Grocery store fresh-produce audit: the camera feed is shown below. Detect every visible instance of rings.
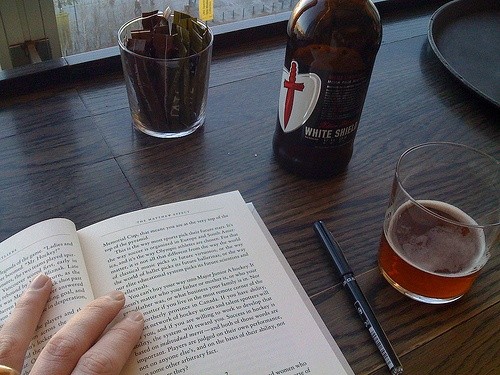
[0,364,21,375]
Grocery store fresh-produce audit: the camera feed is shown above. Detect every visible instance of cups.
[377,140,500,306]
[117,12,214,140]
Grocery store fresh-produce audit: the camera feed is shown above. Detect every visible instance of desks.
[1,0,500,375]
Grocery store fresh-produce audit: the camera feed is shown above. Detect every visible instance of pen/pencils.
[314,220,404,375]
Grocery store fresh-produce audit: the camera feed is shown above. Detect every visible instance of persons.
[0,273,146,375]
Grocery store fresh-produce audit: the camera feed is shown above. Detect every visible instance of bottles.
[272,0,383,180]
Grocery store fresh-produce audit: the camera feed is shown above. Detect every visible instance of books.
[0,189,354,375]
